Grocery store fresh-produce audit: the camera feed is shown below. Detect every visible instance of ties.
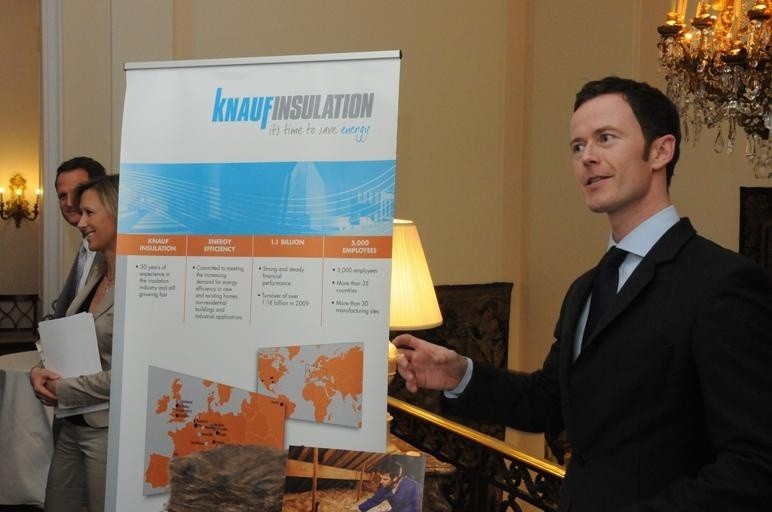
[71,244,87,296]
[583,246,626,345]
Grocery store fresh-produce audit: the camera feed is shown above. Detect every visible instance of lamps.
[0,172,43,229]
[654,1,772,179]
[389,217,443,388]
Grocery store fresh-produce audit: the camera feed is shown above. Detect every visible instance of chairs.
[0,295,40,352]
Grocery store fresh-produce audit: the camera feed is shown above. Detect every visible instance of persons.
[350,459,421,512]
[390,76,772,511]
[29,173,119,512]
[53,155,106,321]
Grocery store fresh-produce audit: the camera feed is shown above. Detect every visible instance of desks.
[0,347,53,507]
